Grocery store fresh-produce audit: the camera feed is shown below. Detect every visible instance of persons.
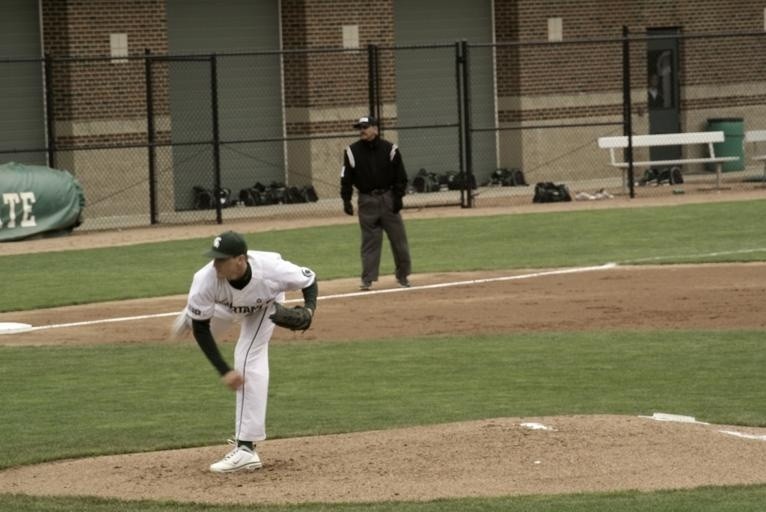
[171,226,318,475]
[340,118,411,291]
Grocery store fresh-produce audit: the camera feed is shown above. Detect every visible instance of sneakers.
[210,445,262,473]
[575,188,614,200]
[343,199,353,216]
[393,195,402,214]
[360,280,371,288]
[396,273,410,287]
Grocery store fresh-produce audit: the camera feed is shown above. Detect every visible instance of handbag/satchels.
[412,167,477,192]
[189,181,318,210]
[532,182,572,202]
[489,168,529,187]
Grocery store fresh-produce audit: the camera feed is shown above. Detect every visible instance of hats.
[201,231,247,259]
[352,117,377,127]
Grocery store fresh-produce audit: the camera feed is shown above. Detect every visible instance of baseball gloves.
[270,301,310,329]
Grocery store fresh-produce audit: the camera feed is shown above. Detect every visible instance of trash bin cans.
[708,117,745,172]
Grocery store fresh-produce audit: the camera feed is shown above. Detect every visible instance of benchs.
[598,131,738,196]
